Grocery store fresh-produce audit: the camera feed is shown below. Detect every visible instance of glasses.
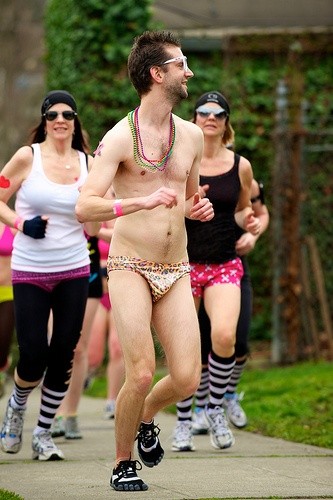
[158,56,189,71]
[43,111,78,121]
[195,105,229,119]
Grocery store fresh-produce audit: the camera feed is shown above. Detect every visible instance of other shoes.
[51,414,82,439]
[223,393,247,429]
[103,401,115,419]
[192,406,210,434]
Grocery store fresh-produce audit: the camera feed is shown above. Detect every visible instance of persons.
[0,91,101,461]
[171,91,263,450]
[74,31,214,491]
[0,180,270,439]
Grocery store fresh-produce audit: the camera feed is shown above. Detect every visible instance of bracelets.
[14,215,23,231]
[127,105,176,173]
[113,199,123,217]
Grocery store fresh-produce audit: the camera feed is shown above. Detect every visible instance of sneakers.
[202,401,235,450]
[109,451,149,491]
[31,429,65,461]
[171,420,195,451]
[1,398,24,454]
[134,418,164,468]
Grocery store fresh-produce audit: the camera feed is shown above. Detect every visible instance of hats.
[193,91,230,115]
[40,89,78,113]
